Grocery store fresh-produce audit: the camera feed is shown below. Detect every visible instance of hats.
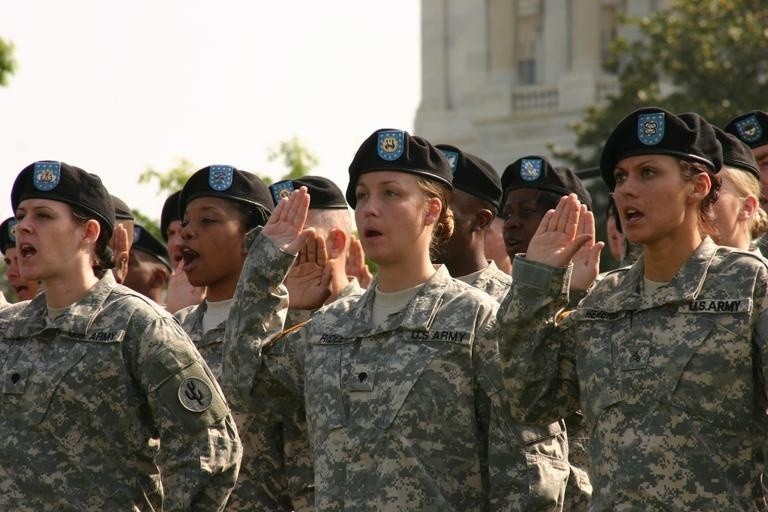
[131,224,173,273]
[599,106,724,192]
[495,154,593,219]
[160,190,182,243]
[177,164,275,219]
[109,194,134,220]
[722,109,768,150]
[10,160,116,258]
[345,128,456,211]
[0,217,18,256]
[434,144,503,210]
[709,124,762,181]
[268,175,348,210]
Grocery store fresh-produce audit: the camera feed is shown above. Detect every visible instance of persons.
[497,109,767,511]
[113,166,289,511]
[567,126,768,306]
[496,152,591,275]
[1,160,244,511]
[270,173,366,311]
[604,200,624,259]
[160,188,205,314]
[283,143,511,324]
[220,127,571,512]
[125,223,169,309]
[723,109,768,219]
[1,216,40,301]
[92,192,133,256]
[345,201,510,290]
